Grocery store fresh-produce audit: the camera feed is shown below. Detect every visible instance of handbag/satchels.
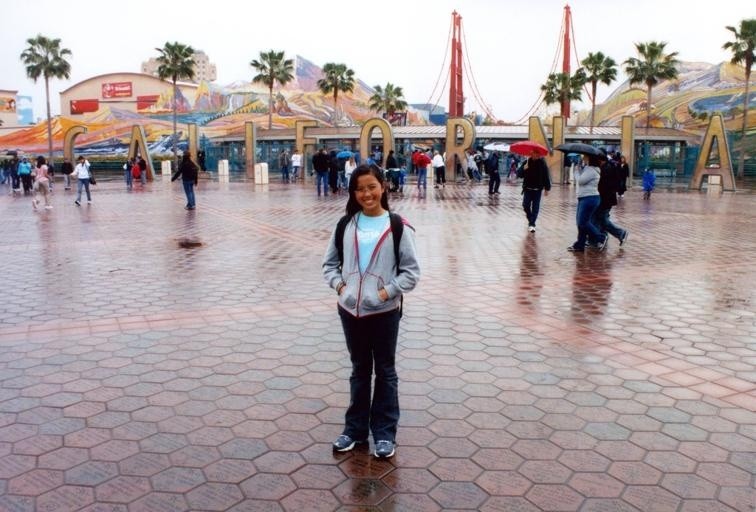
[89,175,96,184]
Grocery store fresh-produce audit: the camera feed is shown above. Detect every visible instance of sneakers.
[528,226,535,232]
[373,439,396,458]
[332,435,368,452]
[567,231,628,251]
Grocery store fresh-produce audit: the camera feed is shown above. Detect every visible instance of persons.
[322,162,421,458]
[126,157,136,189]
[462,148,523,194]
[197,148,207,172]
[69,156,92,207]
[280,141,447,198]
[517,150,552,231]
[137,153,147,187]
[562,152,631,251]
[171,150,198,209]
[0,152,74,210]
[641,166,656,200]
